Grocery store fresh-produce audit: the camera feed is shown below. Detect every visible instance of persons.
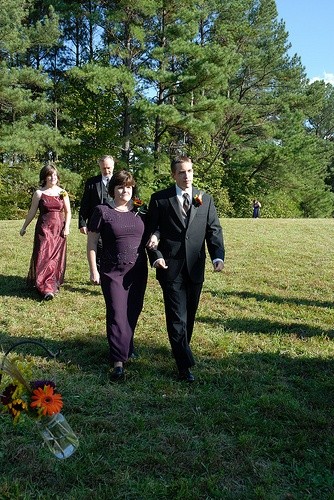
[252,199,261,218]
[145,156,225,381]
[78,155,115,271]
[20,165,72,301]
[86,170,160,378]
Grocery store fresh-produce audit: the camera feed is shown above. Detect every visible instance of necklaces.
[113,200,130,211]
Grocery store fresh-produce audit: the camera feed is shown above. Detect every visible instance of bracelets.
[22,229,26,231]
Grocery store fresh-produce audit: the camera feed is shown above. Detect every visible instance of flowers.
[193,192,204,207]
[59,190,66,197]
[0,352,63,425]
[133,197,147,215]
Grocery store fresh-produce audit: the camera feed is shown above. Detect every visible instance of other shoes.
[44,293,54,301]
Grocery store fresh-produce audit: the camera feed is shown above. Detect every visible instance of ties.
[182,192,190,216]
[105,178,109,188]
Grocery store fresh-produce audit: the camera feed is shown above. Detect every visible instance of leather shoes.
[178,367,195,381]
[110,362,125,382]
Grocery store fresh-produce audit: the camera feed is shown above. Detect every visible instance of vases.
[35,413,79,459]
